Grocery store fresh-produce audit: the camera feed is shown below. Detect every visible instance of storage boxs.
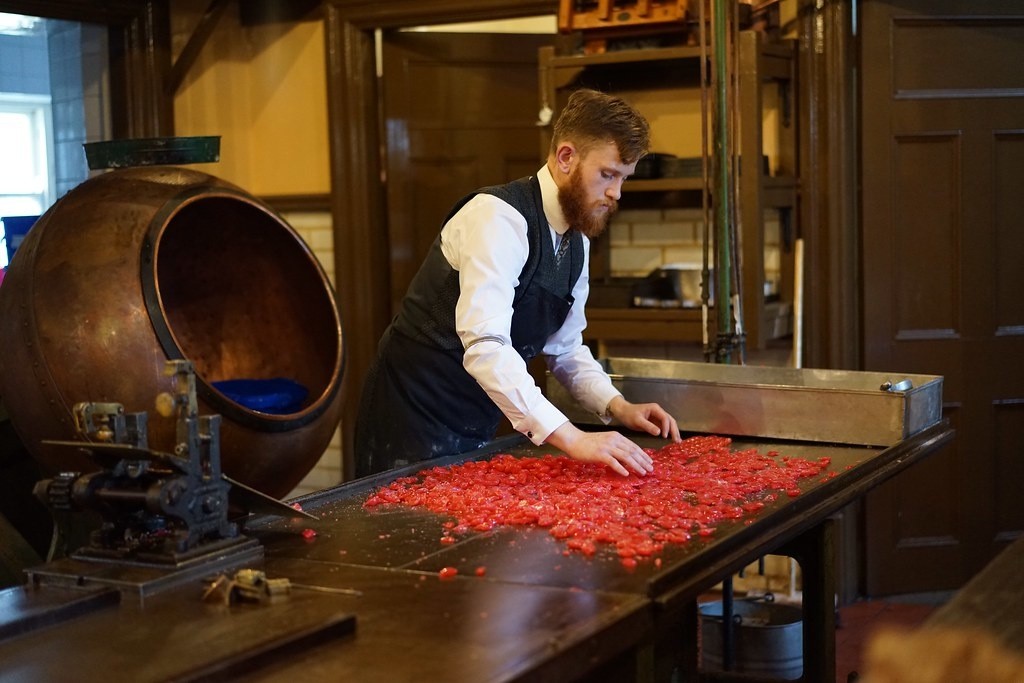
[543,357,943,447]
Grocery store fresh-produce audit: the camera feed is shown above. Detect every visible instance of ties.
[556,228,575,268]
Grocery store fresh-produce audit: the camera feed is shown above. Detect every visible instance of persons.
[352,90,682,477]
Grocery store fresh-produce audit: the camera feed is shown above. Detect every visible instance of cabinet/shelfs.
[535,31,795,352]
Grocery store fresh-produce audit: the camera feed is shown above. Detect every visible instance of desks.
[0,402,957,683]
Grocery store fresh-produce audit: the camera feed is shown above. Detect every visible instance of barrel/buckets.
[696,593,803,683]
[0,164,346,552]
